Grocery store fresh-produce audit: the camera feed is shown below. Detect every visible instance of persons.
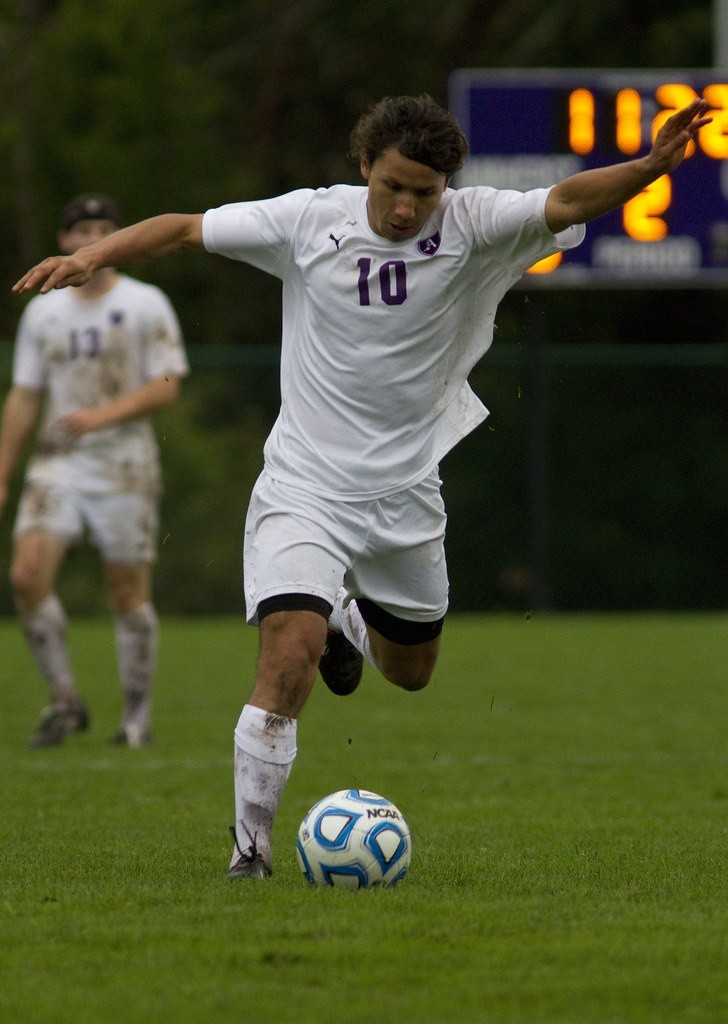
[0,194,189,747]
[12,94,712,882]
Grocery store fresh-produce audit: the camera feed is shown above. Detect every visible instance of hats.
[59,192,125,232]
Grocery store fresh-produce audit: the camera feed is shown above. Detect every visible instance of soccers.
[296,790,412,887]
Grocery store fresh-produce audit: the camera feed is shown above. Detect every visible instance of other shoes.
[28,699,90,749]
[105,731,153,749]
[227,819,272,883]
[318,627,364,696]
